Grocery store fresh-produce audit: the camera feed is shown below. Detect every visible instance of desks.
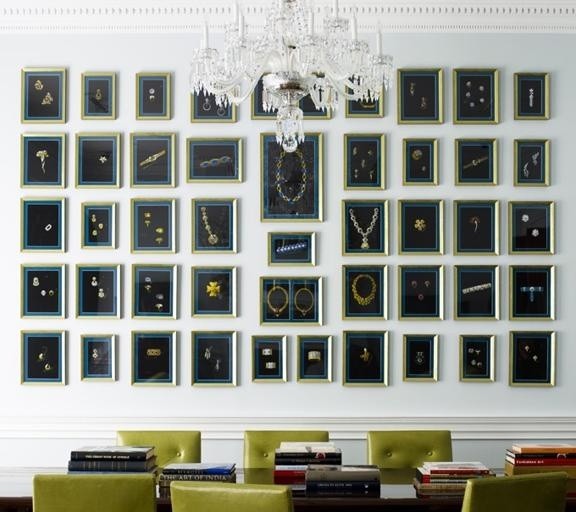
[1,464,505,510]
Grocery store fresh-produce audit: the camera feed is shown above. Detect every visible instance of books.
[503,441,575,500]
[159,462,236,499]
[412,461,496,500]
[274,442,343,497]
[68,446,158,474]
[305,465,381,499]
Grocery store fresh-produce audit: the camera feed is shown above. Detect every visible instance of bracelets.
[200,155,233,169]
[140,150,165,168]
[276,243,307,252]
[519,286,544,301]
[462,283,493,295]
[459,155,490,171]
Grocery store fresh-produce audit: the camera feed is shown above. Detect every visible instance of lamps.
[187,2,398,156]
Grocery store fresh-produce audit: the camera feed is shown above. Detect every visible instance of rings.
[262,349,273,356]
[146,348,162,357]
[307,351,324,360]
[265,362,277,370]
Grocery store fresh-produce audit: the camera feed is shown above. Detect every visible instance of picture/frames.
[401,137,440,188]
[257,130,326,225]
[187,70,241,124]
[507,263,558,323]
[399,332,441,384]
[130,262,180,321]
[190,196,240,255]
[455,332,496,384]
[257,274,326,328]
[506,329,558,388]
[128,130,178,190]
[19,69,68,124]
[450,69,502,127]
[395,198,447,258]
[73,262,123,321]
[73,130,123,191]
[78,332,118,383]
[249,70,334,122]
[126,196,179,256]
[249,333,290,384]
[510,70,552,122]
[340,263,390,322]
[185,136,245,184]
[343,74,387,121]
[395,262,447,323]
[451,262,502,323]
[512,137,552,189]
[189,263,241,321]
[19,130,68,191]
[78,199,119,252]
[396,70,446,126]
[78,70,118,122]
[133,70,174,122]
[266,230,318,268]
[19,328,67,388]
[342,132,388,193]
[18,194,68,254]
[129,328,179,389]
[294,333,335,384]
[339,196,392,259]
[451,198,502,258]
[454,136,500,188]
[188,329,239,388]
[505,199,558,258]
[340,329,391,389]
[18,261,69,322]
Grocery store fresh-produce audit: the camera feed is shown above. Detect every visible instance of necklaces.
[276,146,308,207]
[350,207,379,250]
[351,274,377,307]
[294,287,314,316]
[201,206,226,246]
[268,285,290,318]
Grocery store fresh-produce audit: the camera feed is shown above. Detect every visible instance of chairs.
[459,471,574,511]
[168,478,293,511]
[114,426,203,464]
[363,428,454,469]
[243,429,330,483]
[31,470,158,510]
